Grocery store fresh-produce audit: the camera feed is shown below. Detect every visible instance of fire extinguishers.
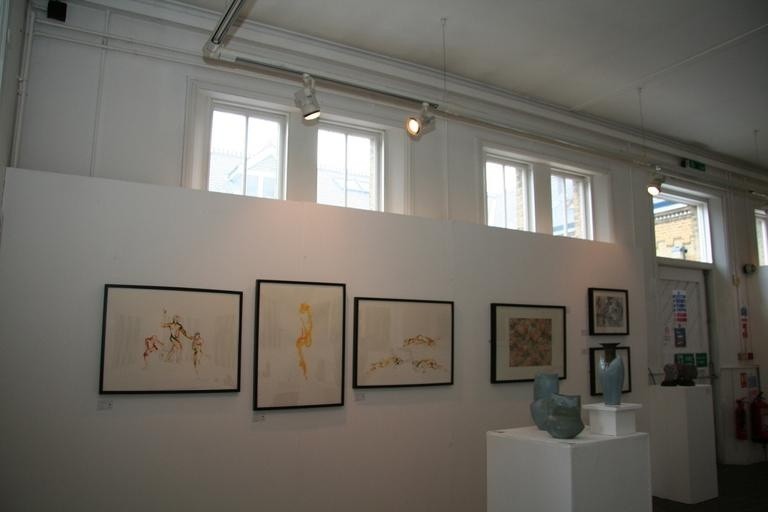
[734,391,768,443]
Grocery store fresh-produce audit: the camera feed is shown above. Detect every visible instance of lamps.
[407,101,434,136]
[647,165,664,196]
[295,72,320,122]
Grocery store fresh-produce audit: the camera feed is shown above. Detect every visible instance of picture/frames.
[252,280,347,411]
[352,296,454,389]
[589,346,631,398]
[588,287,630,338]
[489,301,568,385]
[100,284,243,396]
